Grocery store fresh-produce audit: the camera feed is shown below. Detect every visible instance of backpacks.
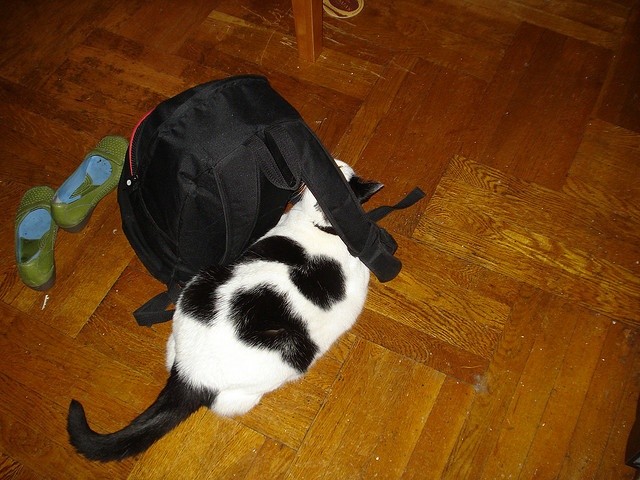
[117,74,428,327]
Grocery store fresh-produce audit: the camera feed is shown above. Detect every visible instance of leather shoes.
[14,187,58,292]
[53,135,129,234]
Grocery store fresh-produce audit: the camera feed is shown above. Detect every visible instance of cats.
[66,159,386,463]
